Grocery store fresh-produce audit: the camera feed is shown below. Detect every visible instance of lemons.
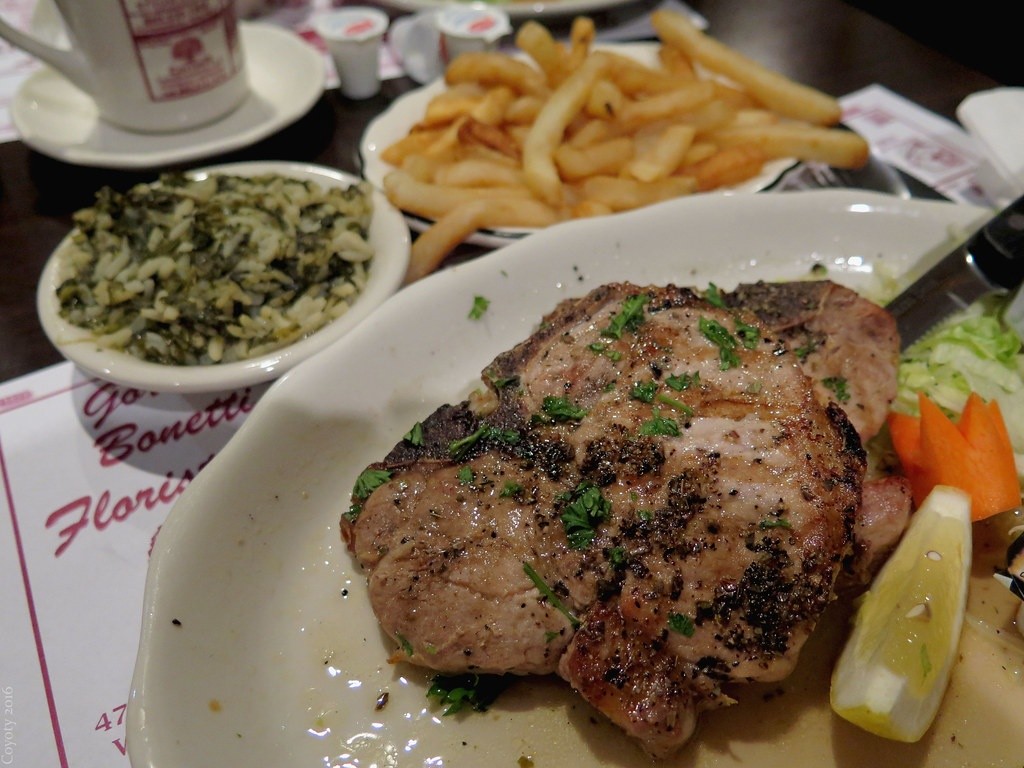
[829,484,972,742]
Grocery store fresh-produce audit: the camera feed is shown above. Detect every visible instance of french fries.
[382,12,871,286]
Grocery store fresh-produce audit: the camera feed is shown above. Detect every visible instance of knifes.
[883,192,1024,367]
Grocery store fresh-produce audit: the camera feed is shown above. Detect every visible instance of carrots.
[887,391,1021,523]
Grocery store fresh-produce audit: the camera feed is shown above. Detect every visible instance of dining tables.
[1,1,1023,764]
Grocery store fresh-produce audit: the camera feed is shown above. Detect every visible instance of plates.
[127,177,1021,766]
[32,157,411,392]
[358,36,814,246]
[10,21,331,171]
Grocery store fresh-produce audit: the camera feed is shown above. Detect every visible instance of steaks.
[339,279,913,761]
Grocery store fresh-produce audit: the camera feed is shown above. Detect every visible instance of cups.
[1,0,254,137]
